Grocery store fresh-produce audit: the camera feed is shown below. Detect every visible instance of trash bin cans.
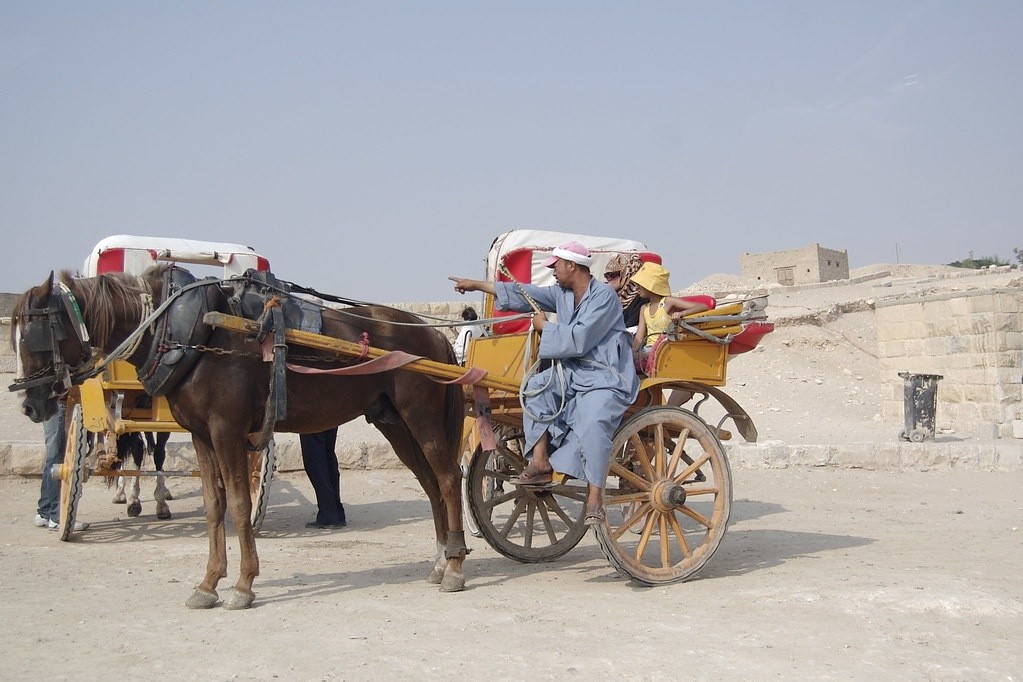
[897,371,944,442]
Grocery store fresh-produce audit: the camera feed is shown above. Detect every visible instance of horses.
[10,263,468,611]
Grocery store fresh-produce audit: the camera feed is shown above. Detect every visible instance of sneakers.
[48,518,90,530]
[35,515,49,526]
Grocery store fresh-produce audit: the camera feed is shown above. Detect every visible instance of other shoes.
[340,521,346,526]
[304,520,340,530]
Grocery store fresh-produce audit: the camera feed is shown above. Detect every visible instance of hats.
[630,262,671,296]
[543,240,591,268]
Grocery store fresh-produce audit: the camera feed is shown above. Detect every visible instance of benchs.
[648,295,717,377]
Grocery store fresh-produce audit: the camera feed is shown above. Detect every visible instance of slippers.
[509,466,552,484]
[584,506,606,525]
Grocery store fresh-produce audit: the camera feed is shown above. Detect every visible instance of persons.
[603,253,650,328]
[450,307,490,366]
[34,401,92,531]
[448,241,641,524]
[629,261,709,374]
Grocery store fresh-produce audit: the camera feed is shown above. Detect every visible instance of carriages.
[8,264,775,611]
[462,229,662,538]
[51,236,275,543]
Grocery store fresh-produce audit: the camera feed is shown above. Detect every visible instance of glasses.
[604,272,620,281]
